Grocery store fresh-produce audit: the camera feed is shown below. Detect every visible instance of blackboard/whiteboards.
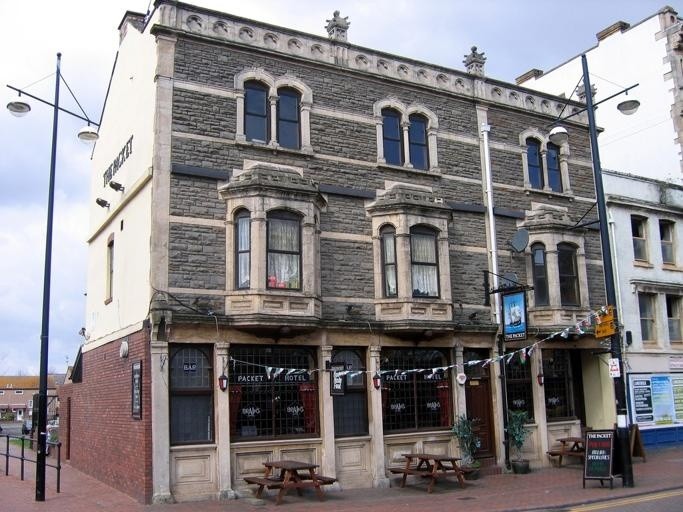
[131,360,142,421]
[584,429,614,480]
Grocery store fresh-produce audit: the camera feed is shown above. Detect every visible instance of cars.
[19,413,61,448]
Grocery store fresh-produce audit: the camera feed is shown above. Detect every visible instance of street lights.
[543,48,646,486]
[4,47,110,502]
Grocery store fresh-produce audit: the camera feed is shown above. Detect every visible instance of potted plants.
[502,406,539,474]
[449,410,483,482]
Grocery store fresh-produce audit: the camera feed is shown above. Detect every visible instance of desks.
[398,454,464,496]
[556,437,588,470]
[256,458,324,505]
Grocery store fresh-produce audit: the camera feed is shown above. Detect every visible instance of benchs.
[241,476,287,493]
[387,464,440,478]
[546,450,587,459]
[299,473,337,487]
[438,462,475,478]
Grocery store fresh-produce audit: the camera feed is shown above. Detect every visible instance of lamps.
[537,372,546,386]
[218,371,228,393]
[372,370,382,391]
